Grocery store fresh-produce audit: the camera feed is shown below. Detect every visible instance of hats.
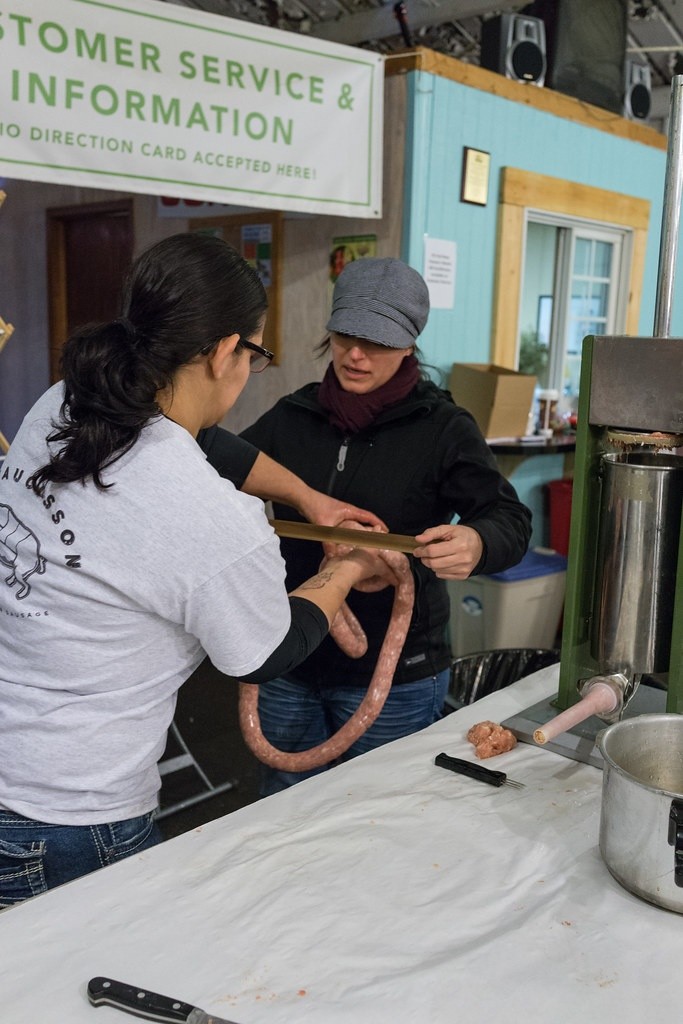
[325,256,430,349]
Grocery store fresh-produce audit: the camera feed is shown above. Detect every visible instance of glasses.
[202,334,275,374]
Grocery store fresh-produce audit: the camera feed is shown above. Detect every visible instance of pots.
[595,714,683,915]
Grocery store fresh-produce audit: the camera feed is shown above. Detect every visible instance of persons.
[0,232,398,911]
[236,258,536,799]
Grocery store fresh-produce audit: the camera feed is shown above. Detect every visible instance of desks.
[0,661,683,1024]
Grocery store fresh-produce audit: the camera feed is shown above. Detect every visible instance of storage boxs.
[451,363,538,439]
[448,545,568,658]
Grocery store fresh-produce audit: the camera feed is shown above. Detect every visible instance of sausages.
[237,521,414,771]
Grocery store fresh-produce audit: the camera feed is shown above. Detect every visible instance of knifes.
[87,977,240,1024]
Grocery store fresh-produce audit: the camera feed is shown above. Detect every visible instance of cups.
[538,389,558,439]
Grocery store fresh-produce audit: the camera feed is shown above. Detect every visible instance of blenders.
[500,335,683,770]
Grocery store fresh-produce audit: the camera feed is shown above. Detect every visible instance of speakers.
[622,60,653,123]
[519,0,627,114]
[480,14,548,88]
